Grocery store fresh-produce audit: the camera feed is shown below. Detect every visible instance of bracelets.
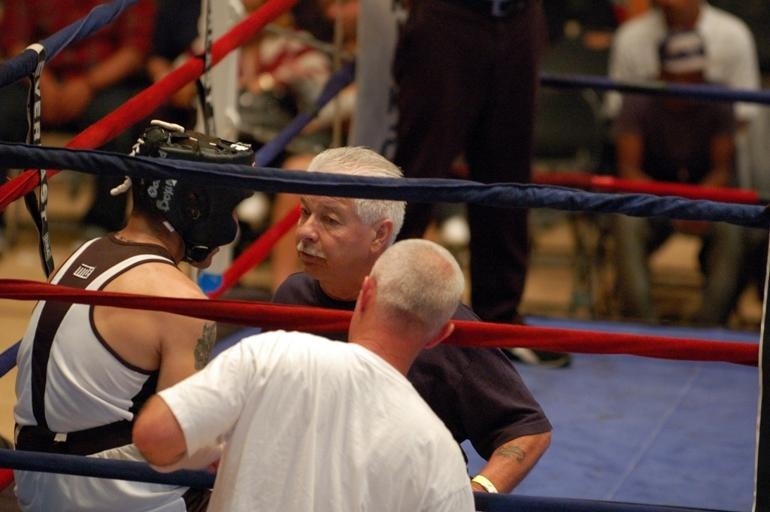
[472,475,498,494]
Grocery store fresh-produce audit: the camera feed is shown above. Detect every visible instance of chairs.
[531,91,612,318]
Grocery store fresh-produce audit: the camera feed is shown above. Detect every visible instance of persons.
[13,119,261,512]
[1,3,769,364]
[260,146,553,494]
[132,238,475,512]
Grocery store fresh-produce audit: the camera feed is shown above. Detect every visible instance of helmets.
[128,120,256,262]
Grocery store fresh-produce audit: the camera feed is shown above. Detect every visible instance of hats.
[660,30,708,76]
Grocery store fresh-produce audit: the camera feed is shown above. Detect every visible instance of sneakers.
[502,347,571,368]
